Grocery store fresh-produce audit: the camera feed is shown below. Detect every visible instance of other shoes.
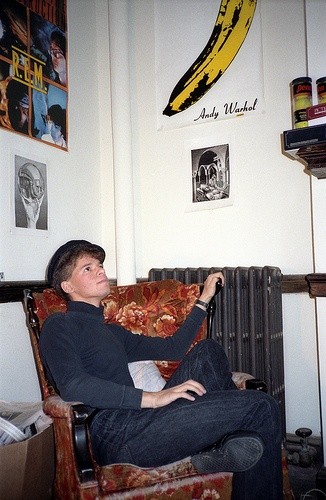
[192,431,264,474]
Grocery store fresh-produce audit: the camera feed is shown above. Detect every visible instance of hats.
[47,240,106,285]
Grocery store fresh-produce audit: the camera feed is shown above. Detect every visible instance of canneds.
[290,77,313,128]
[315,76,326,104]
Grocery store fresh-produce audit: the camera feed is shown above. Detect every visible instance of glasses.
[48,48,66,59]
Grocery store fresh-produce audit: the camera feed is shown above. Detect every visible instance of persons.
[0,1,66,148]
[38,239,283,500]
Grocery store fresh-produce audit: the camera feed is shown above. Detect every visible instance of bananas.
[162,0,257,114]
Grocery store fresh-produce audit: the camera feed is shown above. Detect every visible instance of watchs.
[193,297,209,309]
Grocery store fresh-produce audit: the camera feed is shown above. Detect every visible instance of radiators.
[149,266,287,450]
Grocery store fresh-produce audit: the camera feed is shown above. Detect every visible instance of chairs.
[21,279,292,500]
